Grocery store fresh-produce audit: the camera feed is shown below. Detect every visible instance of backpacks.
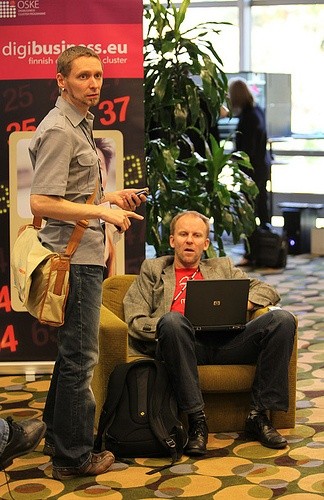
[93,357,188,459]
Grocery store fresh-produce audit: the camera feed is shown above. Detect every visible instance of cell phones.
[127,189,148,203]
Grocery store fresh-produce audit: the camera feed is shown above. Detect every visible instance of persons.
[121,210,296,458]
[92,137,112,191]
[227,78,274,268]
[27,46,149,481]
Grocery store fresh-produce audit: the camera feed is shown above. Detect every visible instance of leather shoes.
[52,450,115,480]
[182,410,209,456]
[244,412,288,449]
[43,443,55,455]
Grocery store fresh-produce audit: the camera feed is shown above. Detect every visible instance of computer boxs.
[283,208,316,256]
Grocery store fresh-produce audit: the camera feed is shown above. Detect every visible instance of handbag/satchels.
[11,221,70,328]
[242,224,288,270]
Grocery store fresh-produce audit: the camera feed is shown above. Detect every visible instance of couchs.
[84,276,298,432]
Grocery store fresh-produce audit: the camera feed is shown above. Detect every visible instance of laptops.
[184,278,251,333]
